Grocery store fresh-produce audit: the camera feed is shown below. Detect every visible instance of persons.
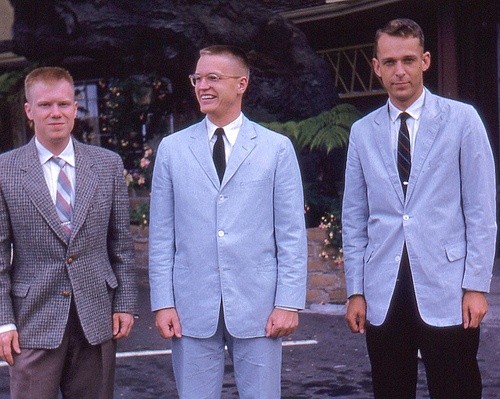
[148,45,308,399]
[342,19,498,399]
[0,67,138,399]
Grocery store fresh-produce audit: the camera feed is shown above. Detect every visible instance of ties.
[213,128,226,186]
[52,156,75,241]
[397,113,411,199]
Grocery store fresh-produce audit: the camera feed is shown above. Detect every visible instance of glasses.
[189,73,241,87]
